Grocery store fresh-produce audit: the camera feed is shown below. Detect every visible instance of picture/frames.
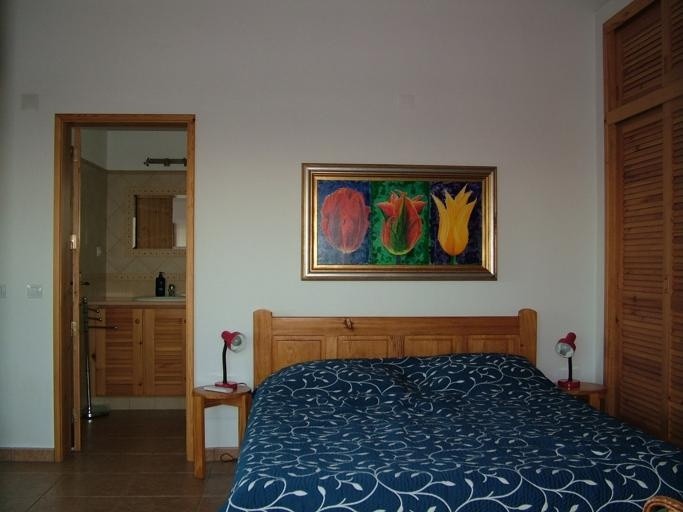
[298,163,498,283]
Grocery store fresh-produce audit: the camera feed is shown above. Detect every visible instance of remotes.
[204,384,234,394]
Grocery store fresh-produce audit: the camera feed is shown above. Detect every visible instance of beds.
[226,309,681,507]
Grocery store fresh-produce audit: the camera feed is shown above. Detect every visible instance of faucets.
[168,284,175,297]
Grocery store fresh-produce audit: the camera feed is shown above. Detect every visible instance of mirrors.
[133,193,187,251]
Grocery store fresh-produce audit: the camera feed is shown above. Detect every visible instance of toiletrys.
[155,271,166,296]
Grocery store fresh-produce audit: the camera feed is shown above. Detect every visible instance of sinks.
[133,295,186,301]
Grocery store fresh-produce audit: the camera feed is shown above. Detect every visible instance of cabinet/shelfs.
[88,306,185,398]
[598,1,683,445]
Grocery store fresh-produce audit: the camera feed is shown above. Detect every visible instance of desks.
[191,383,250,480]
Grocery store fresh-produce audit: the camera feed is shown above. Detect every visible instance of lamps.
[214,328,247,391]
[554,332,582,389]
